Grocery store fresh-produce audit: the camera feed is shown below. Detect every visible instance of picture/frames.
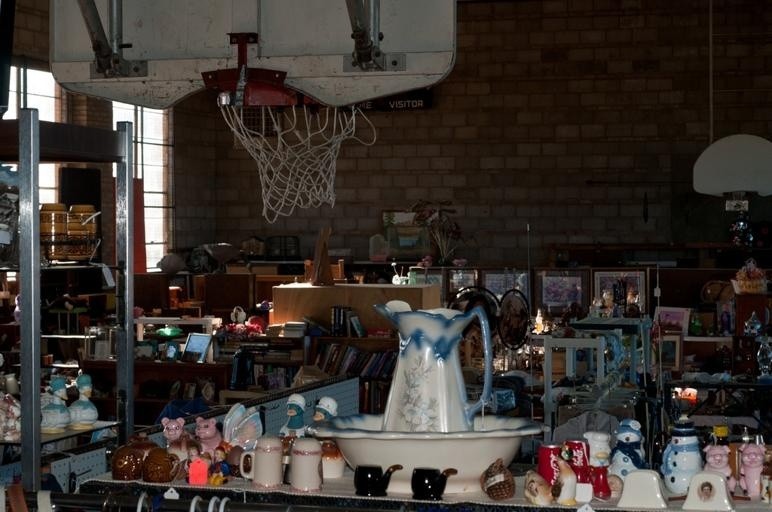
[182,332,212,363]
[409,266,651,316]
[653,306,691,336]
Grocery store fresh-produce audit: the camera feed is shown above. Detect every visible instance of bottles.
[311,227,335,286]
[4,373,19,395]
[84,325,117,360]
[40,203,98,260]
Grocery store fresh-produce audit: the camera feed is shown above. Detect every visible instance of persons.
[719,310,731,333]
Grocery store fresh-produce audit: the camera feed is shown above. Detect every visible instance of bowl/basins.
[304,415,552,494]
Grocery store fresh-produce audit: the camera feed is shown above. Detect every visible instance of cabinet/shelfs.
[539,334,605,441]
[254,275,305,325]
[1,108,133,495]
[234,333,400,417]
[193,272,254,325]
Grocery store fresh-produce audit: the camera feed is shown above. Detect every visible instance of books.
[221,304,401,415]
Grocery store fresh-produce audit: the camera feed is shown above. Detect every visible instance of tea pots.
[372,299,493,432]
[113,429,201,482]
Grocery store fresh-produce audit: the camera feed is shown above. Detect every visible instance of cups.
[239,437,345,494]
[407,272,417,285]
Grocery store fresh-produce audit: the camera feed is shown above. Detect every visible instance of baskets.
[730,279,767,294]
